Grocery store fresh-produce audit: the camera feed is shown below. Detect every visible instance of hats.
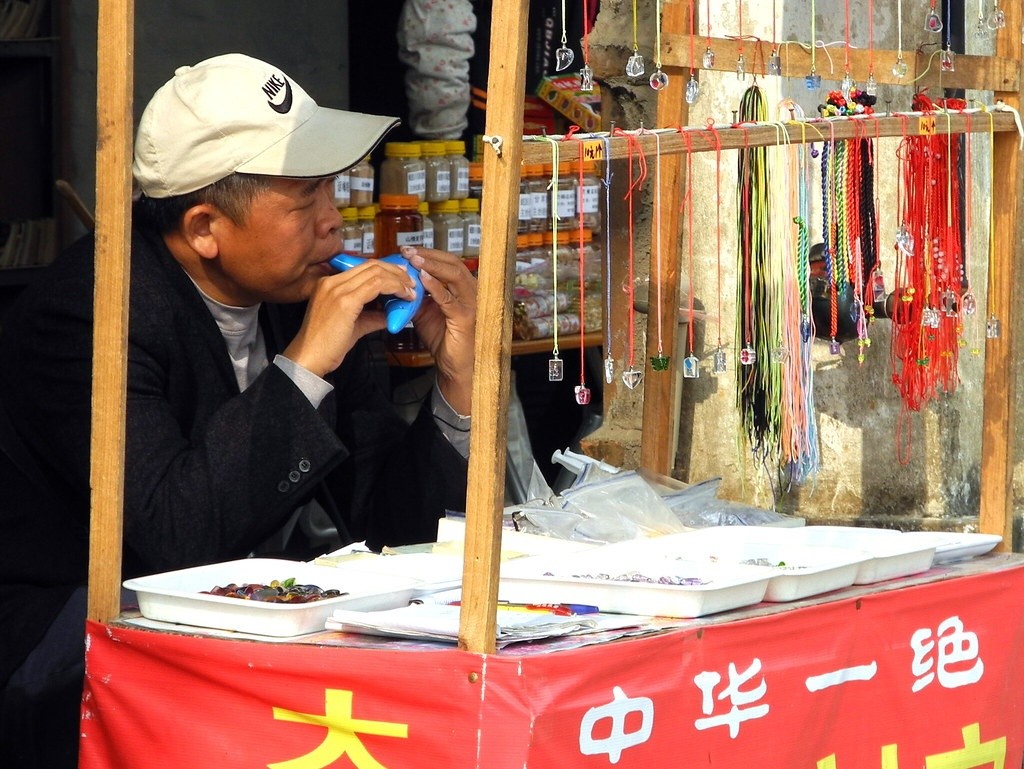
[132,52,400,199]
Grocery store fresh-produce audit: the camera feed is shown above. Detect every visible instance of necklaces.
[535,0,1004,407]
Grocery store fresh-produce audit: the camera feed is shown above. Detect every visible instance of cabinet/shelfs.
[1,0,74,308]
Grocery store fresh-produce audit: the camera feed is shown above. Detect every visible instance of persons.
[0,53,477,769]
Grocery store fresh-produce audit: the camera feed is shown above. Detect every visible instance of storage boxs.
[522,71,602,135]
